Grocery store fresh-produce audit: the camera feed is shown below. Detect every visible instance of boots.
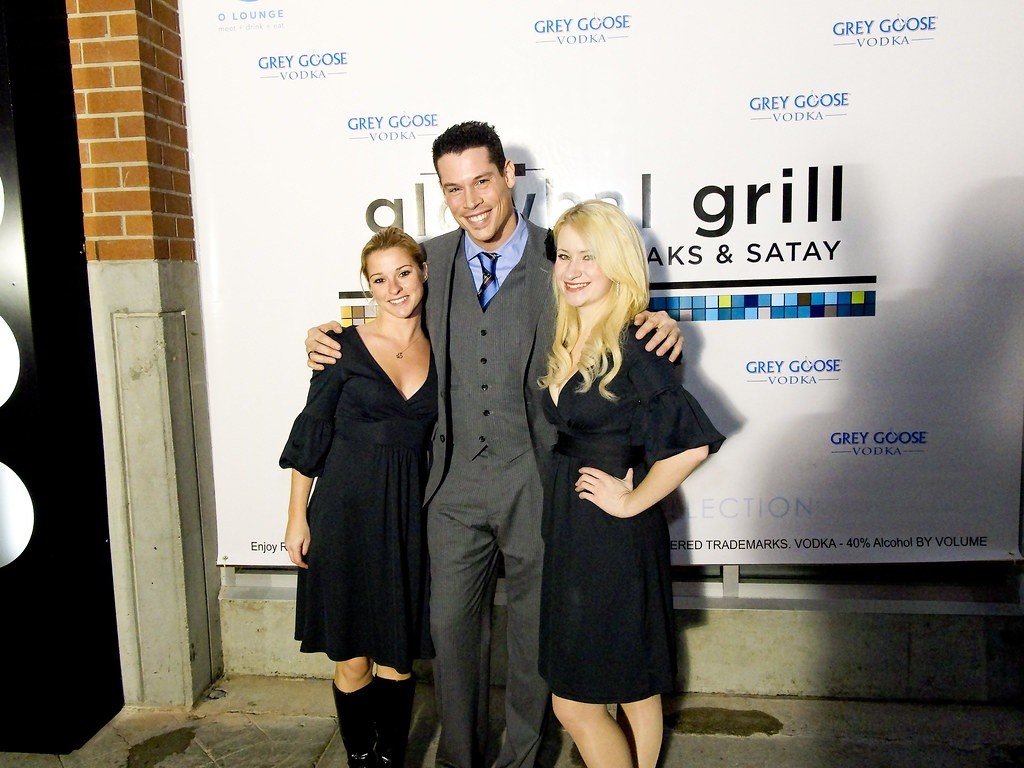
[374,673,417,768]
[332,676,376,768]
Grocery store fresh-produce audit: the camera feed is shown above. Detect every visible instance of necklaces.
[395,334,422,359]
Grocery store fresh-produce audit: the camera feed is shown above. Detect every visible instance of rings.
[308,351,312,358]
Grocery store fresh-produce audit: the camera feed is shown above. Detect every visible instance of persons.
[305,119,683,768]
[538,201,726,768]
[279,224,439,768]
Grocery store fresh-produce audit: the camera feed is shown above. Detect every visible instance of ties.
[477,251,502,313]
